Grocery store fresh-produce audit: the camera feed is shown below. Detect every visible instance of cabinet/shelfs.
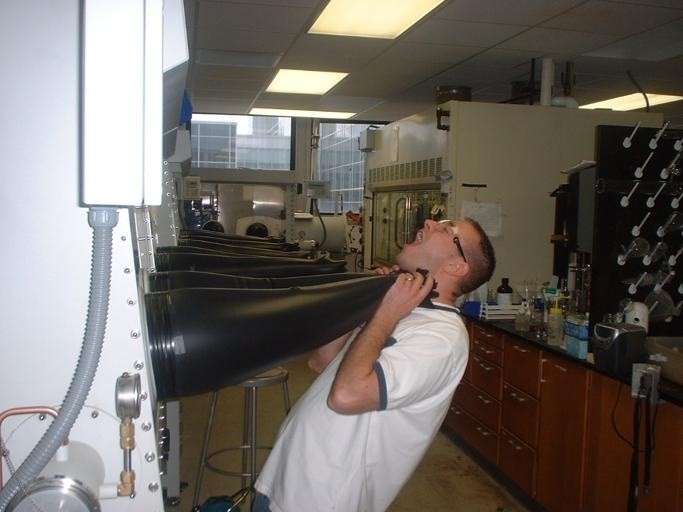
[497,330,543,512]
[439,316,502,486]
[534,346,588,511]
[580,370,682,511]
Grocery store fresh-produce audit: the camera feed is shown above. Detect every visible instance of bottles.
[497,277,569,346]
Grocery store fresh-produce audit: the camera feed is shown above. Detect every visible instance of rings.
[405,278,413,281]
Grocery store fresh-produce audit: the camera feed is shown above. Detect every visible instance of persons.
[246,215,497,511]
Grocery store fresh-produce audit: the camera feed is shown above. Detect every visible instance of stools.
[191,364,290,511]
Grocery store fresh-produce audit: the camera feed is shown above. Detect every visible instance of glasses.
[438,219,467,264]
